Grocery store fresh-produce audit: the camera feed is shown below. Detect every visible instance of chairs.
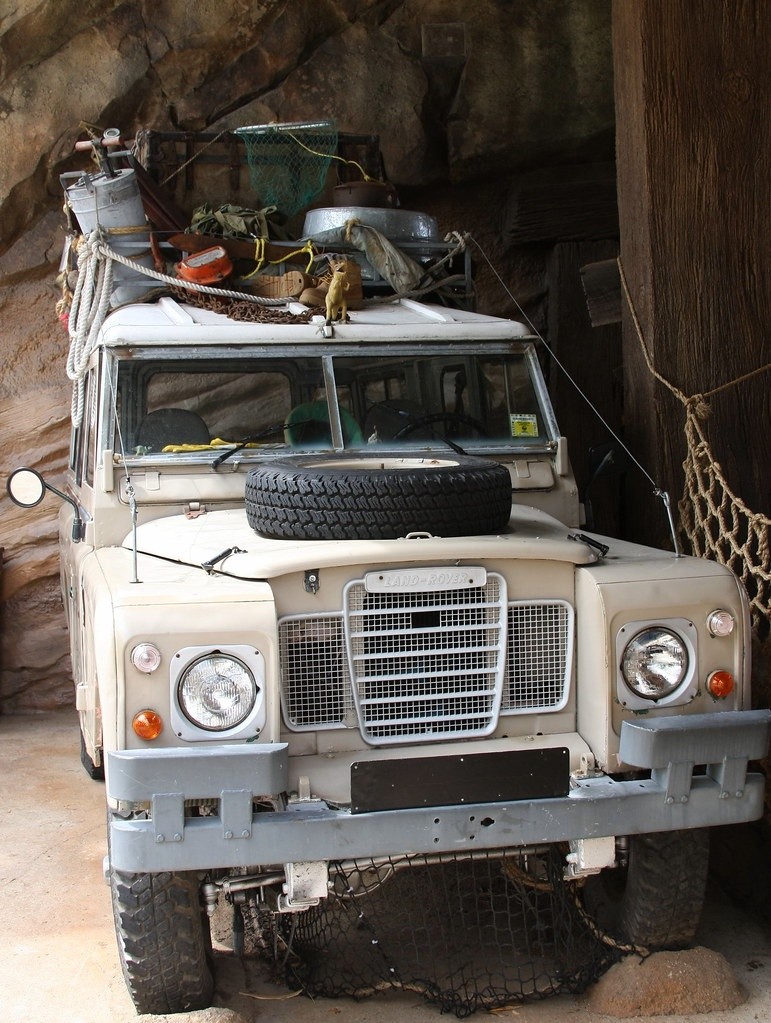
[360,400,434,444]
[134,407,209,453]
[286,397,362,450]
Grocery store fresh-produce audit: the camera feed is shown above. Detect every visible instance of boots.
[300,259,364,308]
[252,271,320,298]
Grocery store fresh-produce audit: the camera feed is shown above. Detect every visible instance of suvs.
[7,297,771,1014]
